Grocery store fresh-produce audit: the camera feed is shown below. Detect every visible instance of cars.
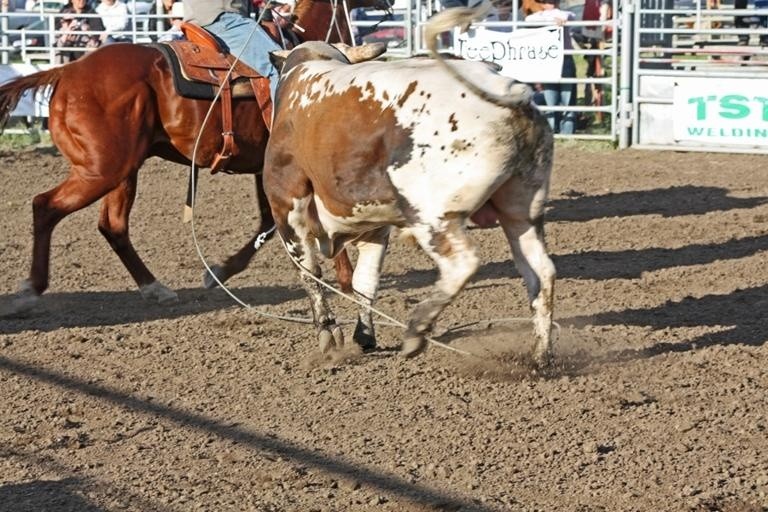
[349,0,607,49]
[672,0,768,47]
[0,0,158,58]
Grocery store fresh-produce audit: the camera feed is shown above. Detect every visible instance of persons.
[183,0,285,133]
[734,0,768,58]
[26,0,184,64]
[490,1,580,136]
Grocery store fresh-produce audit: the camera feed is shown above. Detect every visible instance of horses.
[0,0,395,306]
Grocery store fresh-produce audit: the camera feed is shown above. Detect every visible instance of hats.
[165,2,185,18]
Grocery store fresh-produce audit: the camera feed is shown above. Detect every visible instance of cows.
[262,5,557,378]
